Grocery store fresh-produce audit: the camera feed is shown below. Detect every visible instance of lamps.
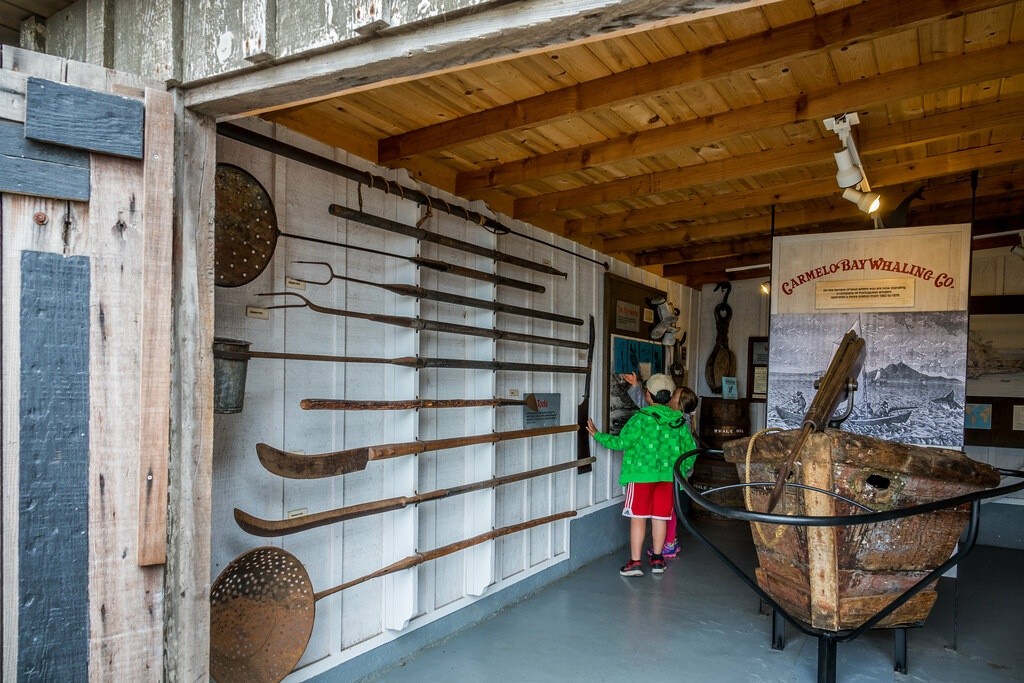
[840,186,881,215]
[833,144,863,189]
[1010,244,1024,260]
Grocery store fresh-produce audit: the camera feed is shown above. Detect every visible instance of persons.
[621,372,700,557]
[586,373,698,577]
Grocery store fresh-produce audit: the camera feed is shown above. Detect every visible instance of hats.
[646,373,676,398]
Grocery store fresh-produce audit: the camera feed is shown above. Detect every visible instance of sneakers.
[620,559,644,577]
[675,539,681,553]
[646,543,676,558]
[650,554,667,573]
